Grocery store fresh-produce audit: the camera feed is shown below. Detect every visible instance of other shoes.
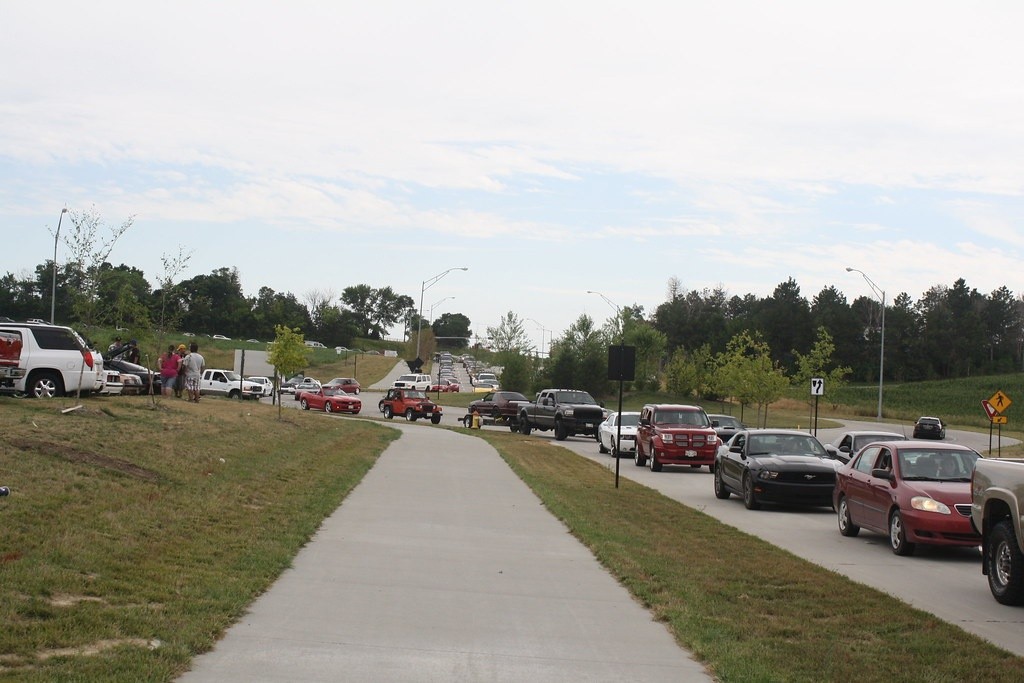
[185,398,193,402]
[193,398,199,403]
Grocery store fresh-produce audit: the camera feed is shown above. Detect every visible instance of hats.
[177,344,186,351]
[130,339,137,345]
[116,337,121,341]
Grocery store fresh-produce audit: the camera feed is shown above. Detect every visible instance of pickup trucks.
[970,456,1022,605]
[196,369,264,401]
[518,388,606,441]
[300,389,362,412]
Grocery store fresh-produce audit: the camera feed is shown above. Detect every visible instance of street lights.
[845,267,887,419]
[50,208,68,323]
[429,297,455,328]
[587,290,625,342]
[416,267,470,367]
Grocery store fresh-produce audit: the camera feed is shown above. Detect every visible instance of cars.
[432,354,499,394]
[824,430,911,467]
[322,377,362,395]
[181,329,379,355]
[712,428,849,512]
[392,373,432,394]
[829,442,988,555]
[246,375,321,395]
[638,404,722,469]
[468,391,531,418]
[99,359,163,396]
[383,387,443,424]
[913,415,946,440]
[705,414,744,445]
[598,410,644,457]
[0,320,106,403]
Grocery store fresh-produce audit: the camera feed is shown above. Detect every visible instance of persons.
[347,380,351,384]
[158,342,206,403]
[128,340,140,366]
[787,440,801,454]
[217,374,225,381]
[307,379,311,382]
[108,336,124,360]
[942,454,958,477]
[91,341,98,349]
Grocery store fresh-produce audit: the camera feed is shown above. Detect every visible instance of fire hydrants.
[471,410,480,429]
